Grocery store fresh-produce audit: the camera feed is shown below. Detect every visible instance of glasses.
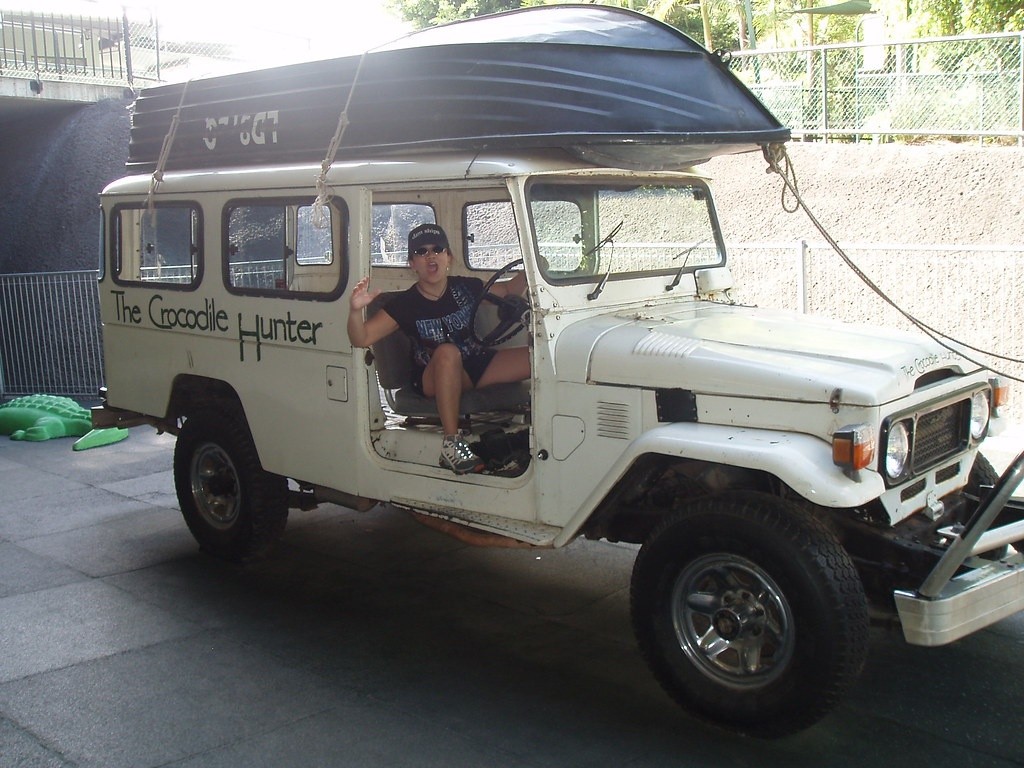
[411,246,447,257]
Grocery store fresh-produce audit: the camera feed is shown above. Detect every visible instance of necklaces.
[418,280,448,298]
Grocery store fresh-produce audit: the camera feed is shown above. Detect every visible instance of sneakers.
[439,433,485,475]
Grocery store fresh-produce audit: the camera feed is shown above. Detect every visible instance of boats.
[124,4,793,170]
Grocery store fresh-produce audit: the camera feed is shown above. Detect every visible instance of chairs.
[373,292,533,434]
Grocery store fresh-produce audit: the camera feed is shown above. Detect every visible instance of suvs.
[90,156,1024,739]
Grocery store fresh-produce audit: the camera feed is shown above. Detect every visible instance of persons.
[346,224,550,475]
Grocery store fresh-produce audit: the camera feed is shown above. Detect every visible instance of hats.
[408,224,449,254]
